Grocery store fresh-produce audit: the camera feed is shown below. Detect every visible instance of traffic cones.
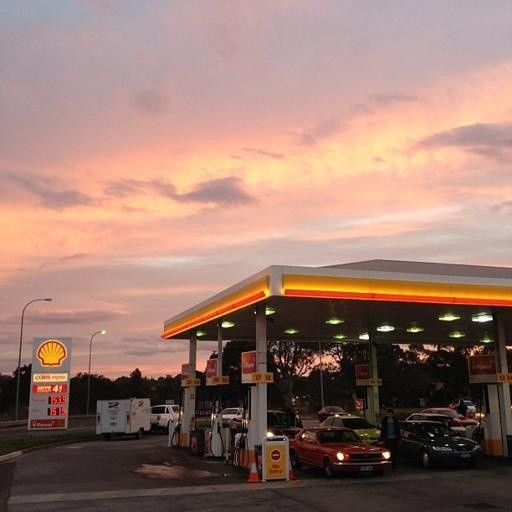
[246,456,263,483]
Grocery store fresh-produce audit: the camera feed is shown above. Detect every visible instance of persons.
[381,409,400,474]
[449,399,468,417]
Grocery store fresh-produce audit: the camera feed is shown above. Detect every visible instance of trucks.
[94,397,152,440]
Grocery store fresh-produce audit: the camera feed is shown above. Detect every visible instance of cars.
[211,407,246,422]
[317,406,351,422]
[405,397,479,437]
[320,415,381,440]
[396,420,481,468]
[267,410,303,437]
[151,404,184,428]
[285,426,392,476]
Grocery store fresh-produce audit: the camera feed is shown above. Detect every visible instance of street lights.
[87,330,106,416]
[16,298,53,421]
